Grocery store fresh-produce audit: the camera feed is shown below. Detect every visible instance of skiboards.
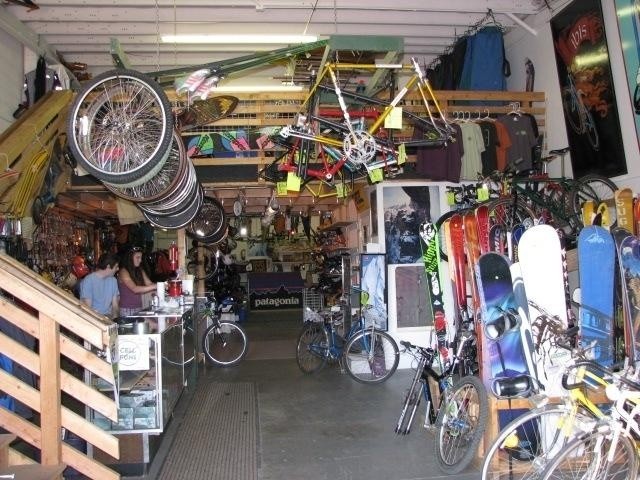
[419,206,503,437]
[583,188,640,236]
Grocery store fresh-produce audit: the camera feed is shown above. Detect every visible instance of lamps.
[158,1,321,43]
[205,85,304,93]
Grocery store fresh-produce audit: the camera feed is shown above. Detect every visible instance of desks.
[82,303,196,435]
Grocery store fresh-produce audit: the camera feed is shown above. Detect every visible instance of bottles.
[179,294,185,306]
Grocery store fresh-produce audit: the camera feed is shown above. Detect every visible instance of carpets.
[156,380,261,480]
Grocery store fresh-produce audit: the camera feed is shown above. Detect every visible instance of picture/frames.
[548,0,640,185]
[359,252,389,332]
[246,272,303,311]
[249,259,267,272]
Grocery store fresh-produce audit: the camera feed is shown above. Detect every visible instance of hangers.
[428,10,508,73]
[448,105,527,128]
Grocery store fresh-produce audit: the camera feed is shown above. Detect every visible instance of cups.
[152,296,159,308]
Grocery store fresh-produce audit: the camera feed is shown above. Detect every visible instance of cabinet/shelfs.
[318,221,352,253]
[271,237,315,265]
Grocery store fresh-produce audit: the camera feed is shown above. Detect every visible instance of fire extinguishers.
[168,240,178,271]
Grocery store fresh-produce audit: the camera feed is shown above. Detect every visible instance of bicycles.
[160,290,249,369]
[296,286,400,384]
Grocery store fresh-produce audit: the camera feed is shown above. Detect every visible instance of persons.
[79,253,120,320]
[117,245,168,317]
[187,262,197,275]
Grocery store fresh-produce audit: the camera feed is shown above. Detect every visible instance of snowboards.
[475,225,640,460]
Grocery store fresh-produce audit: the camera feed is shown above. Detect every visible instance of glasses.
[132,247,142,251]
